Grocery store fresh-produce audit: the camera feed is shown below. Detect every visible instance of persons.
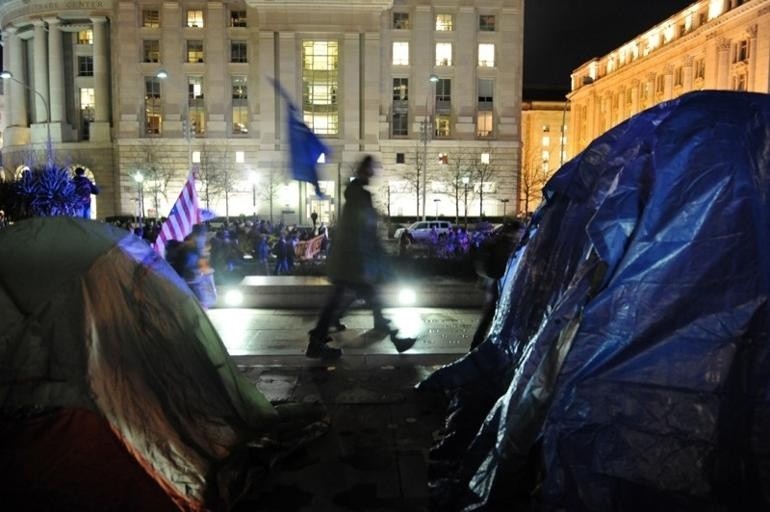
[329,287,391,331]
[71,167,99,220]
[101,212,330,306]
[400,225,493,266]
[306,154,417,358]
[470,220,526,353]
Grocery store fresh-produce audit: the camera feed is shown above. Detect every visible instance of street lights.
[151,71,168,136]
[0,70,52,167]
[560,76,594,168]
[422,75,440,221]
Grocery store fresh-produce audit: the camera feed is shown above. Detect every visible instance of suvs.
[394,220,453,246]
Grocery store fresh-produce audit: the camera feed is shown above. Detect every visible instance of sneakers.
[306,343,341,359]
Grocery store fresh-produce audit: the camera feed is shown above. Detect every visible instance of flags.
[269,77,332,197]
[154,169,215,260]
